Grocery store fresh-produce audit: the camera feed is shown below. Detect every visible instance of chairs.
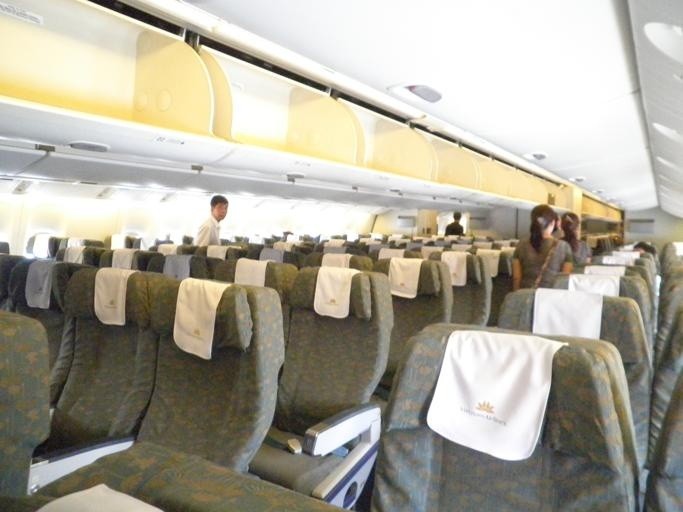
[451,244,476,254]
[106,235,135,249]
[302,252,374,271]
[8,258,92,406]
[377,258,451,399]
[590,255,651,269]
[195,245,248,259]
[358,234,519,246]
[466,248,513,327]
[0,312,51,494]
[602,250,653,261]
[133,280,285,477]
[269,242,312,253]
[377,247,423,260]
[316,242,366,255]
[249,265,393,510]
[246,248,304,267]
[491,288,651,496]
[427,249,490,329]
[552,274,651,345]
[55,239,104,248]
[147,255,209,280]
[149,244,199,255]
[210,260,296,347]
[0,442,350,511]
[56,247,105,266]
[651,239,683,512]
[50,267,162,447]
[412,246,451,259]
[26,235,60,258]
[370,322,640,511]
[100,249,162,271]
[613,240,659,251]
[571,264,653,298]
[133,238,174,249]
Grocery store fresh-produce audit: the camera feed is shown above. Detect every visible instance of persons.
[192,195,228,244]
[512,205,572,291]
[633,242,661,296]
[444,212,464,235]
[558,213,587,265]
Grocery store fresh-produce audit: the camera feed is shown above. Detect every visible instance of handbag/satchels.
[532,237,559,289]
